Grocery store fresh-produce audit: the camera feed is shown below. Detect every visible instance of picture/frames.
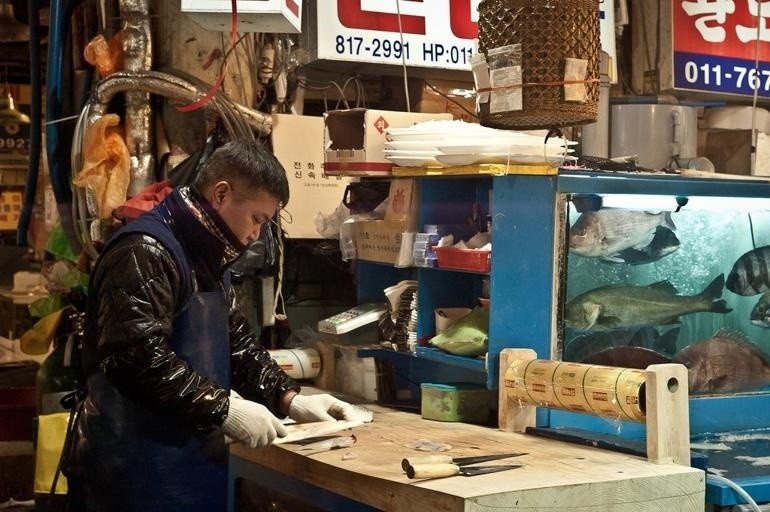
[0,185,26,239]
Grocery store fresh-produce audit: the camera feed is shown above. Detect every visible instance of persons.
[66,136,374,512]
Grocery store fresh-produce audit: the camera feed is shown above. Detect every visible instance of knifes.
[405,464,522,480]
[402,451,528,468]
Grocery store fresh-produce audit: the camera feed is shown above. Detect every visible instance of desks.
[230,386,707,512]
[0,363,41,442]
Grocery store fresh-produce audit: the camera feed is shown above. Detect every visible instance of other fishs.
[582,347,671,370]
[563,328,634,369]
[565,273,733,334]
[727,214,770,296]
[568,207,677,264]
[750,292,770,328]
[628,326,680,355]
[673,328,770,393]
[608,225,681,265]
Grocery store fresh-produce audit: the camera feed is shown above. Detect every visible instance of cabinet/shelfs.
[349,176,567,429]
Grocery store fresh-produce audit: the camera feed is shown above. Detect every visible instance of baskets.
[477,0,600,129]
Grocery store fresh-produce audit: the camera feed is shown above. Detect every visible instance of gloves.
[221,390,288,449]
[289,394,373,422]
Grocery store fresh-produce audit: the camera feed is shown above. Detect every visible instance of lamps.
[0,43,32,124]
[0,0,31,43]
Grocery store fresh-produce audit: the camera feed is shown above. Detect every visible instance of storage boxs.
[416,382,493,424]
[353,178,419,264]
[323,108,454,177]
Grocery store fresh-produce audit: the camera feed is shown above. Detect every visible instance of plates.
[382,125,579,166]
